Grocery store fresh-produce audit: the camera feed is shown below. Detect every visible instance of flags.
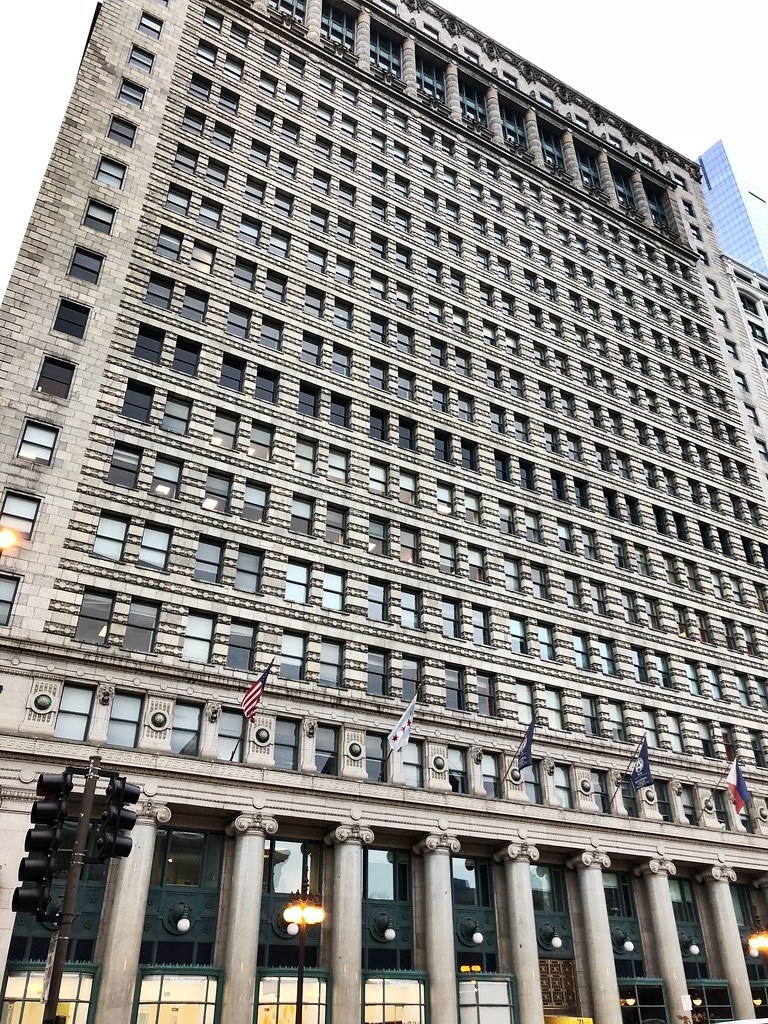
[631,740,654,790]
[726,757,752,816]
[388,693,418,751]
[241,669,268,722]
[518,721,533,769]
